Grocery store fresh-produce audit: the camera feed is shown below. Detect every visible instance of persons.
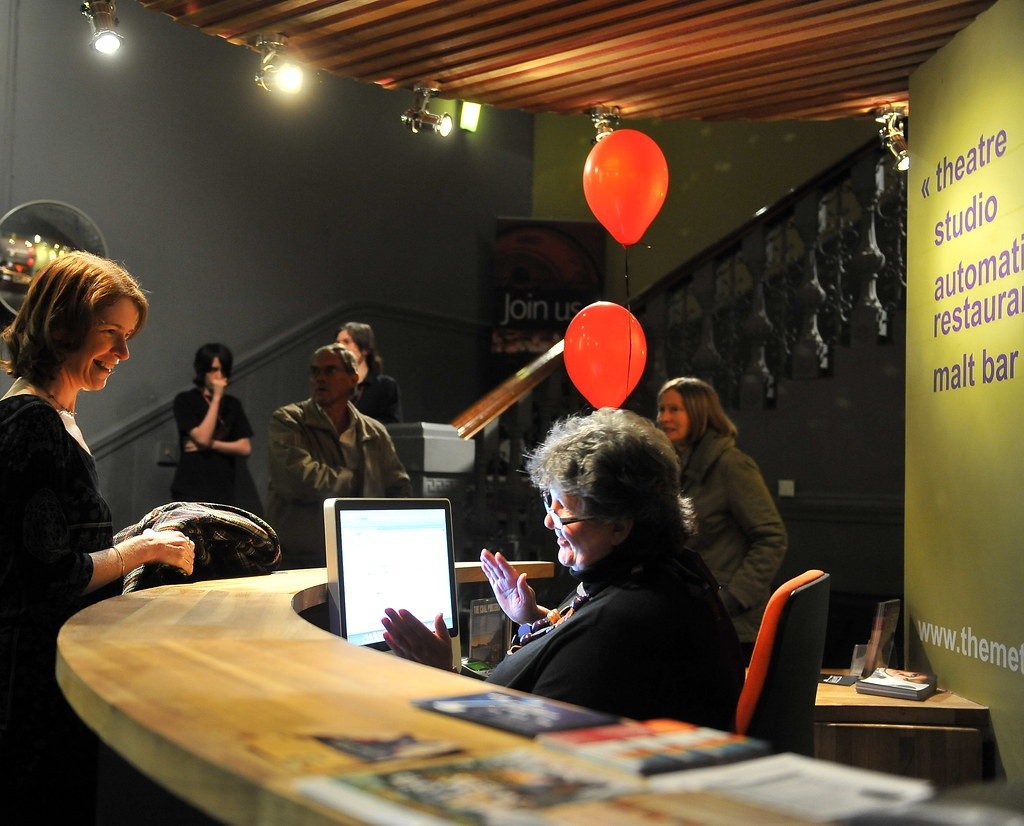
[170,344,263,524]
[0,251,196,826]
[265,343,414,555]
[380,406,749,734]
[656,377,789,676]
[335,321,406,425]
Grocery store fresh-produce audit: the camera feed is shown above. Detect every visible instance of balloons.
[584,126,670,247]
[562,301,648,412]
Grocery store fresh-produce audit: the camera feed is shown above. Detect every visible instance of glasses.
[310,365,348,377]
[544,500,604,530]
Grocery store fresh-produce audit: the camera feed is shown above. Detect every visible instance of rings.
[185,536,190,546]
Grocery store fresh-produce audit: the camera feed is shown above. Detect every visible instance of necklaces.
[44,390,78,417]
[508,588,598,655]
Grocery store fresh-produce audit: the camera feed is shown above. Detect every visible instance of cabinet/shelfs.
[814,669,990,785]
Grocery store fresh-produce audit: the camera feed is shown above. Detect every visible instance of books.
[860,598,938,702]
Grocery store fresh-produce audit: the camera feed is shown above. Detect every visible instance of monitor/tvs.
[324,497,461,674]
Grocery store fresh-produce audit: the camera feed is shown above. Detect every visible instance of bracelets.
[112,546,125,577]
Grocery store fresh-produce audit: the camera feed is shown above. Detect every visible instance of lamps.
[82,1,125,57]
[399,83,456,138]
[255,32,305,95]
[875,112,909,172]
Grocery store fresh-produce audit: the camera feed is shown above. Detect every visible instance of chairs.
[734,568,828,750]
